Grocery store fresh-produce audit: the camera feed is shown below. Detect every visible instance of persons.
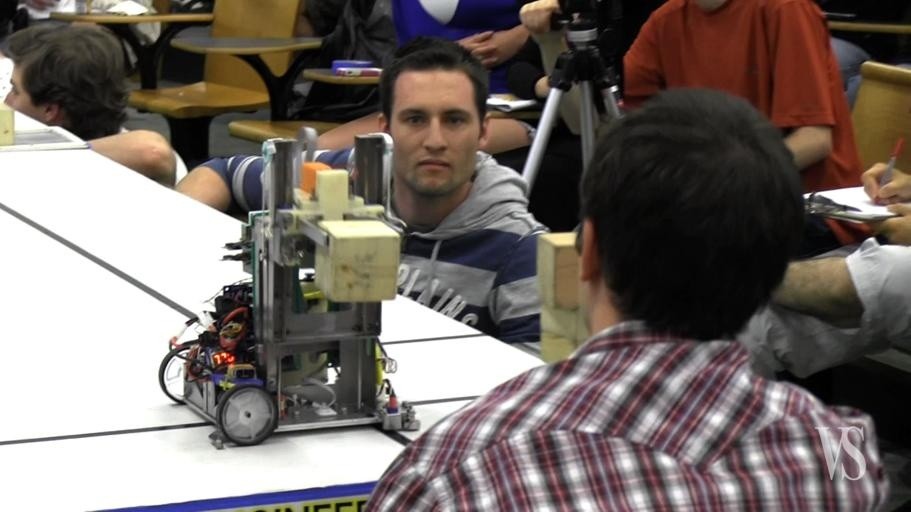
[729,161,911,380]
[317,0,548,159]
[1,21,188,192]
[518,0,864,194]
[15,0,167,91]
[172,36,552,362]
[363,85,890,511]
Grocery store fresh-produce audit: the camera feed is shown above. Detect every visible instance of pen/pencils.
[879,137,906,188]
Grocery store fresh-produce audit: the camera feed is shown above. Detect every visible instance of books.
[0,125,90,152]
[485,93,540,114]
[802,186,911,225]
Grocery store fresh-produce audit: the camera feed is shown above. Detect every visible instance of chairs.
[129,2,301,171]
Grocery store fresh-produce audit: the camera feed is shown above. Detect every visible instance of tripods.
[517,52,631,211]
